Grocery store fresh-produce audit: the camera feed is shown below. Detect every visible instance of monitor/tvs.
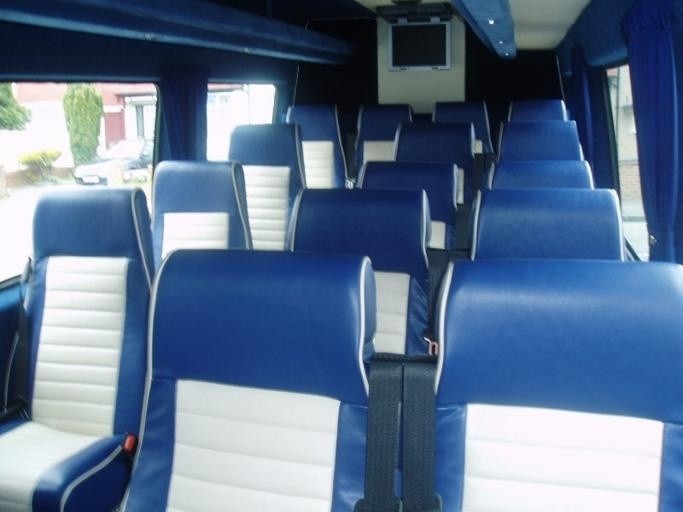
[388,22,451,71]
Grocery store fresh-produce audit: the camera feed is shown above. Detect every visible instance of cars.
[74,136,152,187]
